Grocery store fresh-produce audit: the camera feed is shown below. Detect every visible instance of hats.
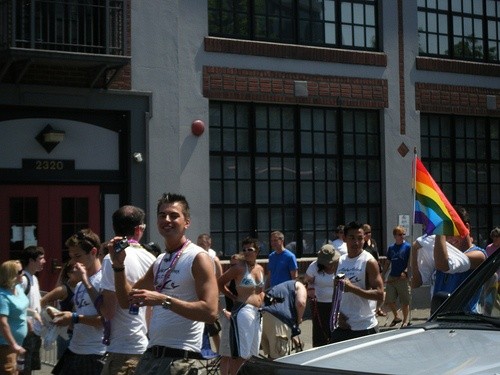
[317,244,340,264]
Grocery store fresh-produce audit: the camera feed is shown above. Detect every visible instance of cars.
[237,247,500,375]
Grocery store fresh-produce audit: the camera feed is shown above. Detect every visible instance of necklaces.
[152,238,190,293]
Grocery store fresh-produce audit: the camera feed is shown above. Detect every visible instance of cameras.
[113,235,130,252]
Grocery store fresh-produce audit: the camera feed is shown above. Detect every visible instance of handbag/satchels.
[329,280,352,338]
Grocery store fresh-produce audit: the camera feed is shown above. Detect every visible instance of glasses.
[365,230,373,235]
[399,234,405,236]
[134,224,146,231]
[18,269,23,274]
[242,248,255,252]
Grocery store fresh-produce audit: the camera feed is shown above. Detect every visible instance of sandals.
[378,310,387,316]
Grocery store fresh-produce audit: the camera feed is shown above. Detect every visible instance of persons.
[486,227,500,257]
[19,246,46,375]
[42,192,222,375]
[383,226,412,328]
[410,205,488,313]
[265,231,298,289]
[218,237,266,360]
[260,276,308,360]
[0,260,33,375]
[304,221,384,347]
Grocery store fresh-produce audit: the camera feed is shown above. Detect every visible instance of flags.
[412,157,470,238]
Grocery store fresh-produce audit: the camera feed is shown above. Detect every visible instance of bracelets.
[94,295,103,306]
[72,313,79,323]
[162,296,171,309]
[113,266,125,272]
[221,308,226,313]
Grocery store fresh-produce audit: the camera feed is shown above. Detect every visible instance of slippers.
[389,319,402,327]
[400,322,410,327]
